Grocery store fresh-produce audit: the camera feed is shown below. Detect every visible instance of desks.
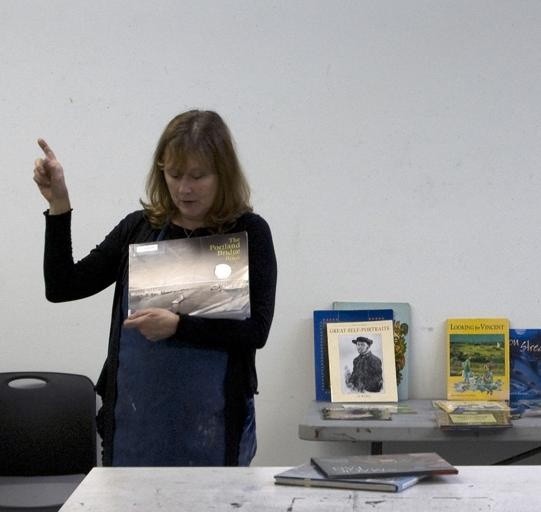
[297,397,541,466]
[58,466,541,511]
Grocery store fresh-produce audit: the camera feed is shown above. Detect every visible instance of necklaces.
[178,213,202,238]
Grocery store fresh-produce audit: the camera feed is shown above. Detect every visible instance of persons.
[32,109,278,466]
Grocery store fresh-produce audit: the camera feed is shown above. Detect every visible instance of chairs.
[1,371,96,511]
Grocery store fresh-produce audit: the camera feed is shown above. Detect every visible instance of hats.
[352,337,374,344]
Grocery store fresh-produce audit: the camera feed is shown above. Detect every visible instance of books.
[273,452,458,492]
[313,301,541,431]
